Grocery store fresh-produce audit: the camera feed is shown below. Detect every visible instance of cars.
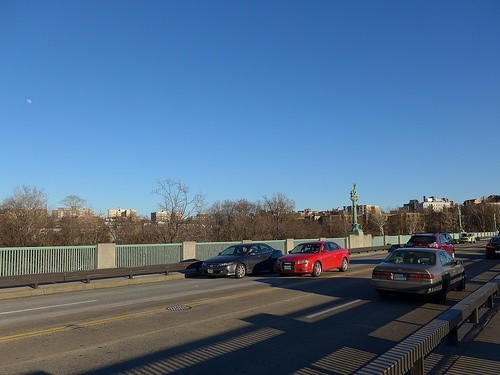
[486,236,500,258]
[371,248,466,300]
[276,241,350,276]
[441,233,454,246]
[202,243,282,277]
[404,234,455,259]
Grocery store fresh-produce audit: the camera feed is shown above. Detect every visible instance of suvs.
[459,232,476,244]
